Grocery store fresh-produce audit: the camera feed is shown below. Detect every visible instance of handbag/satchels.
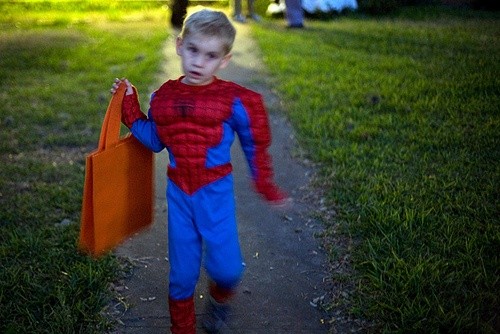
[76,80,156,259]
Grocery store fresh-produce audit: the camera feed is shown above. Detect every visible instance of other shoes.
[246,14,262,23]
[233,15,245,23]
[199,292,232,333]
[287,24,304,29]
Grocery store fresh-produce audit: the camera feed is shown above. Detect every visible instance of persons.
[109,9,294,334]
[234,0,304,29]
